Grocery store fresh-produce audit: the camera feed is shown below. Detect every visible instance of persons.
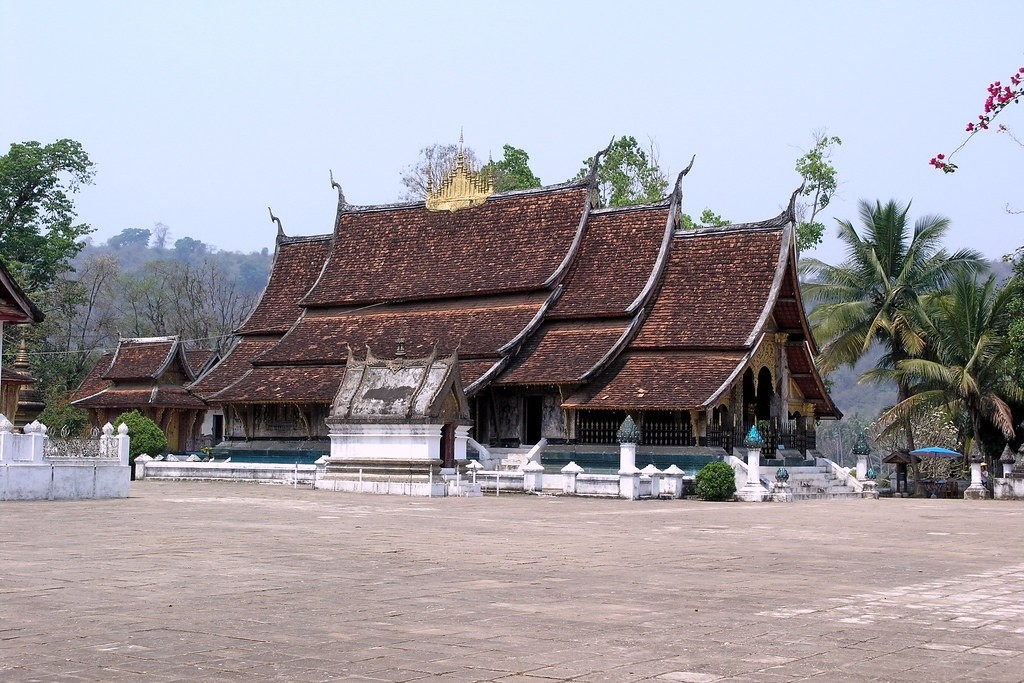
[981,466,988,487]
[947,473,955,490]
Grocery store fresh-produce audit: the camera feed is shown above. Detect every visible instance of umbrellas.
[909,446,963,477]
